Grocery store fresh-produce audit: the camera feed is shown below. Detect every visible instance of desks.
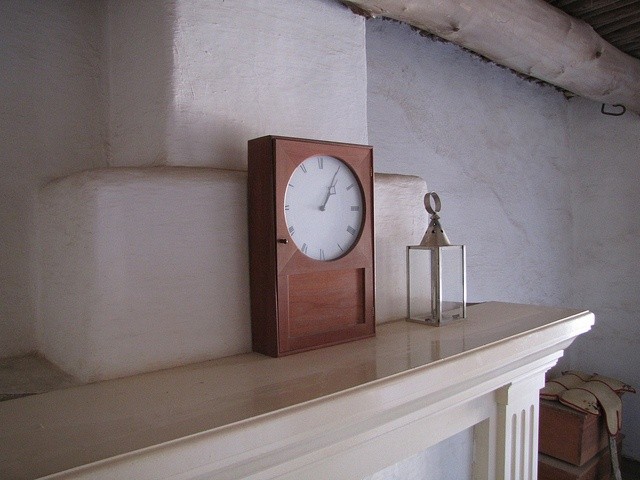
[0,299,597,480]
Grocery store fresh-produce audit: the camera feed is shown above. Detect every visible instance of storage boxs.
[538,432,626,480]
[538,400,620,466]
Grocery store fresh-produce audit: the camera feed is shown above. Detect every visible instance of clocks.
[247,134,377,358]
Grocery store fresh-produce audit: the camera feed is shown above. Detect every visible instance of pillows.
[540,370,592,399]
[560,374,636,418]
